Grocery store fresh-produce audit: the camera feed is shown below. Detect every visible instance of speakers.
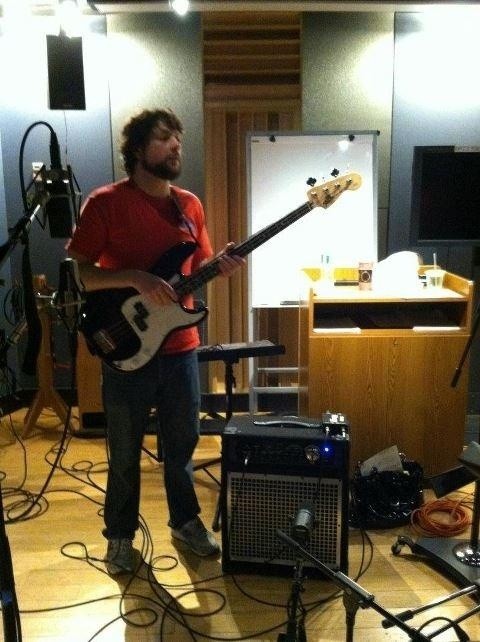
[221,414,352,581]
[46,34,86,111]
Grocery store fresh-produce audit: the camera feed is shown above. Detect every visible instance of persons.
[65,106,246,576]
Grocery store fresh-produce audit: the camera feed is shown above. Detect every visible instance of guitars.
[79,167,363,374]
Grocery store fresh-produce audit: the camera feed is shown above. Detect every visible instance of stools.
[192,340,286,530]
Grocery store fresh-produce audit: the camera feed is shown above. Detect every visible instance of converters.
[321,412,350,432]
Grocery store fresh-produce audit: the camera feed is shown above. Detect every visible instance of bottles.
[358,261,373,292]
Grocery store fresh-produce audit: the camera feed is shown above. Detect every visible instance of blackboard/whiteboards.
[245,129,379,310]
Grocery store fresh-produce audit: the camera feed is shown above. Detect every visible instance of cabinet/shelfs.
[253,283,475,475]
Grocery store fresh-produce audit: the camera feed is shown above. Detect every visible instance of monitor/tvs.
[410,145,479,245]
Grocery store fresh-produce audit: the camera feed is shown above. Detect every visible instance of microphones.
[293,502,315,535]
[42,169,75,240]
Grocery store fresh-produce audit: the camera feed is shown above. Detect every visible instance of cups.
[425,270,446,290]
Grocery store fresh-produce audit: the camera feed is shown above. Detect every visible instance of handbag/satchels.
[348,456,424,529]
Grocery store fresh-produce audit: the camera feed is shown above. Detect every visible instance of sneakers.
[107,538,136,575]
[171,515,220,557]
